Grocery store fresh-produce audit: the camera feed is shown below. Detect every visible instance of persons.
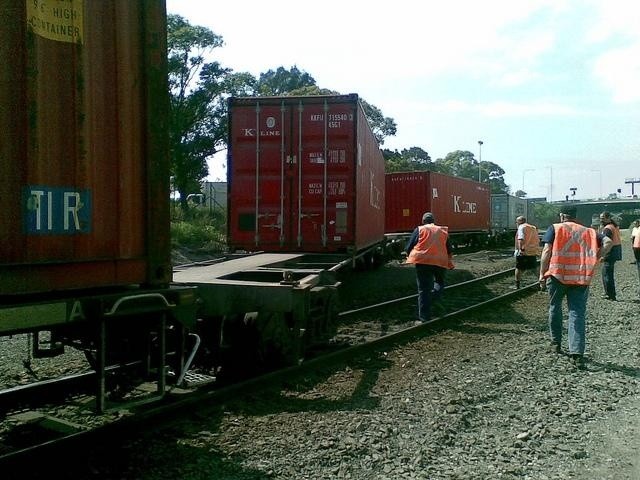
[537,205,614,367]
[508,215,541,290]
[405,212,455,326]
[590,211,640,300]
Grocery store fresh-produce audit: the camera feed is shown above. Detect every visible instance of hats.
[559,205,577,215]
[422,212,434,225]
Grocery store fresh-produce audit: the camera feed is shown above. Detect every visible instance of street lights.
[478,140,483,184]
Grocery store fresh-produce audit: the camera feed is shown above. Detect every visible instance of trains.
[0,0,547,415]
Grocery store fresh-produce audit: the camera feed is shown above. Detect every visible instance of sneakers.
[600,293,616,299]
[414,320,422,325]
[565,354,584,367]
[546,340,560,353]
